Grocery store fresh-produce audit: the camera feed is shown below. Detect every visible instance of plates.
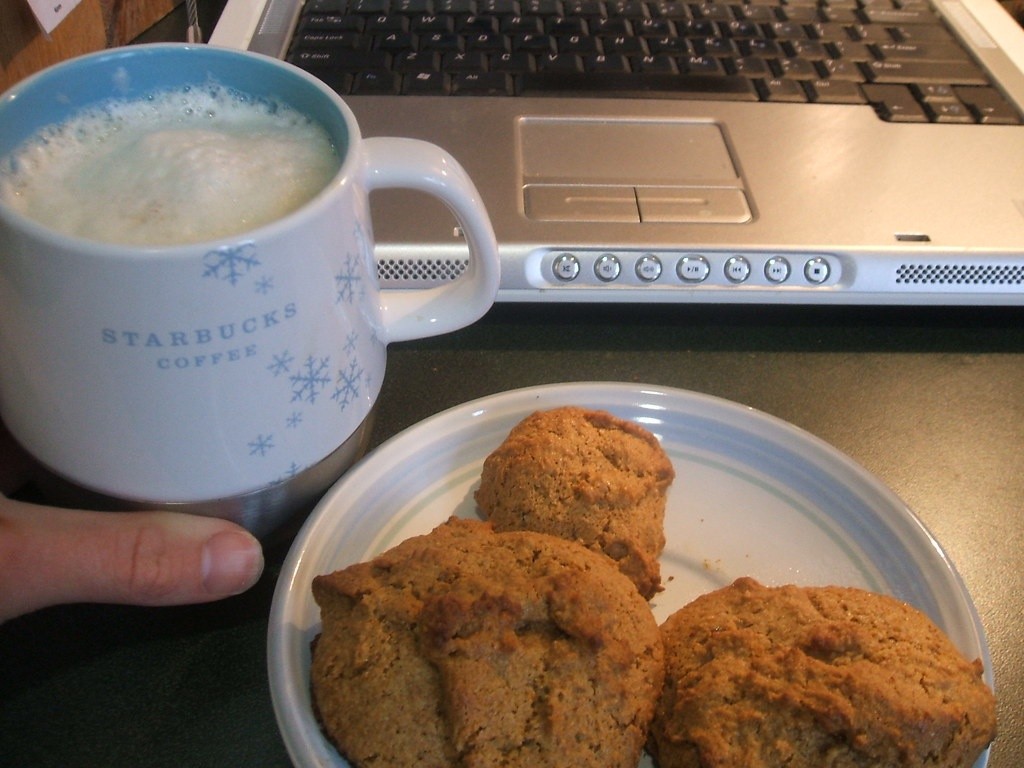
[266,380,993,768]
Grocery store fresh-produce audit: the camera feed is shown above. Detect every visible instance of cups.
[1,44,500,532]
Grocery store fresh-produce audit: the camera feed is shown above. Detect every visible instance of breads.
[470,407,675,604]
[310,516,666,768]
[644,578,1001,768]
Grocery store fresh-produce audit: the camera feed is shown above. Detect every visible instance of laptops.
[206,0,1024,306]
